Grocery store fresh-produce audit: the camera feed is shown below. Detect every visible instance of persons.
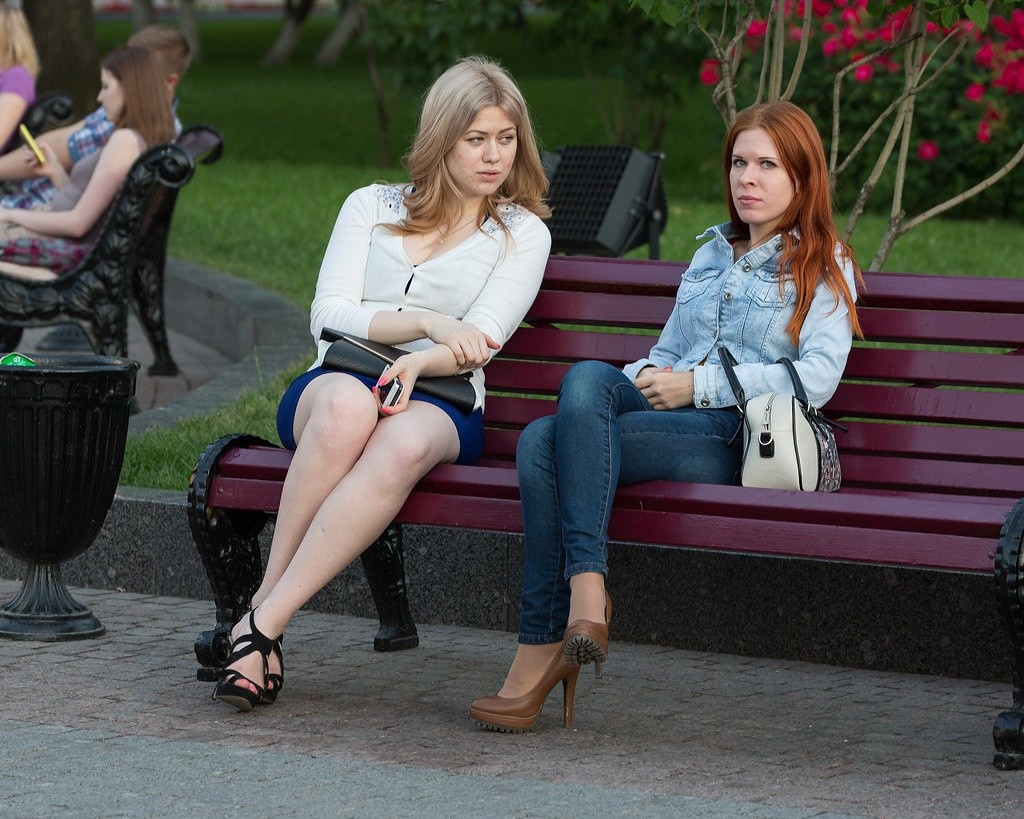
[1,0,192,288]
[193,59,552,711]
[467,97,857,735]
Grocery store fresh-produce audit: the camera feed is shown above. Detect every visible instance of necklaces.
[417,189,478,244]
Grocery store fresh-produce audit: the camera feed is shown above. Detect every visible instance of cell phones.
[374,364,404,409]
[20,124,46,165]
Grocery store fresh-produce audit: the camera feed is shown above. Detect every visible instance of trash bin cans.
[0,354,141,641]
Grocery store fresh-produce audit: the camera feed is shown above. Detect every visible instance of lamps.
[538,144,669,259]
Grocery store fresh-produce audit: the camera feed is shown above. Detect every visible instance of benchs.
[0,126,228,418]
[184,255,1024,771]
[3,87,77,153]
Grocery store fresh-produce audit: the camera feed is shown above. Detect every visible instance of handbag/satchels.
[321,326,476,417]
[740,394,841,493]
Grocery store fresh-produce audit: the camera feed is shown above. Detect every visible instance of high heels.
[213,600,285,713]
[470,641,580,734]
[565,587,612,679]
